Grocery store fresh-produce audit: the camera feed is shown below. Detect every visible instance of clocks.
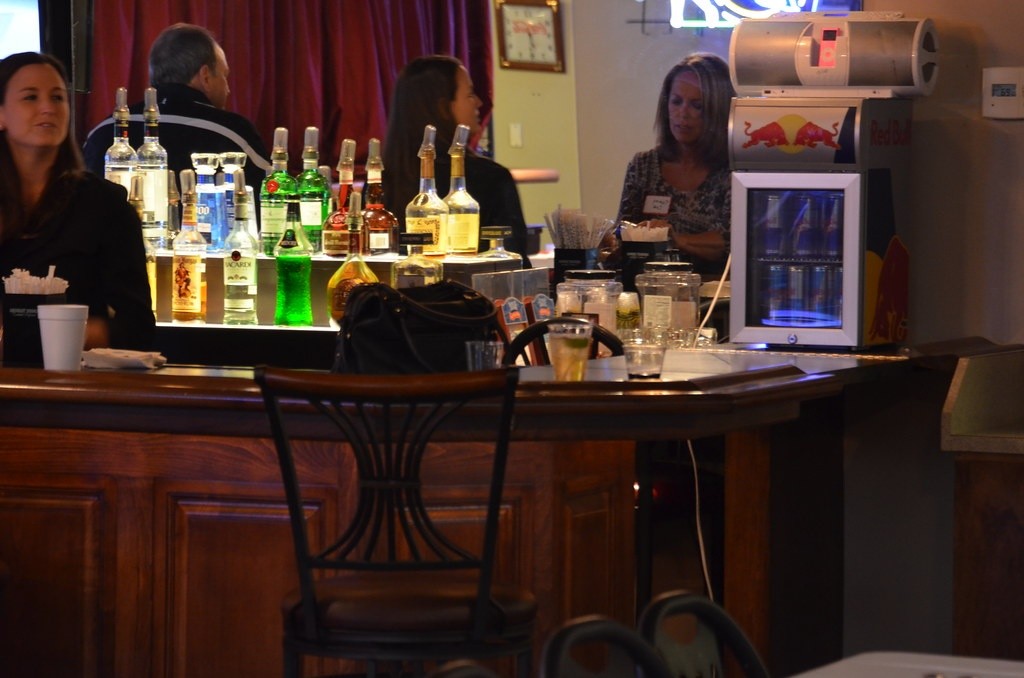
[494,1,566,74]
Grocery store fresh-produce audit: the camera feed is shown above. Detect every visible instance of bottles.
[222,170,259,326]
[260,127,298,257]
[555,268,623,360]
[763,195,841,313]
[172,169,207,324]
[405,125,449,257]
[136,88,169,257]
[295,126,334,252]
[321,138,365,256]
[390,230,443,290]
[216,152,247,251]
[103,87,137,213]
[272,194,313,327]
[635,261,702,340]
[326,191,378,332]
[190,152,220,253]
[440,125,481,257]
[365,139,400,258]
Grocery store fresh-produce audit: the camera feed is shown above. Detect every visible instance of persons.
[0,52,158,367]
[80,22,289,235]
[361,55,529,258]
[596,52,737,260]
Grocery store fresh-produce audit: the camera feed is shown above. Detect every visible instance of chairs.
[254,365,537,678]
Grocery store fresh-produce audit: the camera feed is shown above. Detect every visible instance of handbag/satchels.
[330,276,510,376]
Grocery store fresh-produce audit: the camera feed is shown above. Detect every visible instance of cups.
[621,328,667,381]
[544,323,593,382]
[466,340,503,370]
[37,303,89,371]
